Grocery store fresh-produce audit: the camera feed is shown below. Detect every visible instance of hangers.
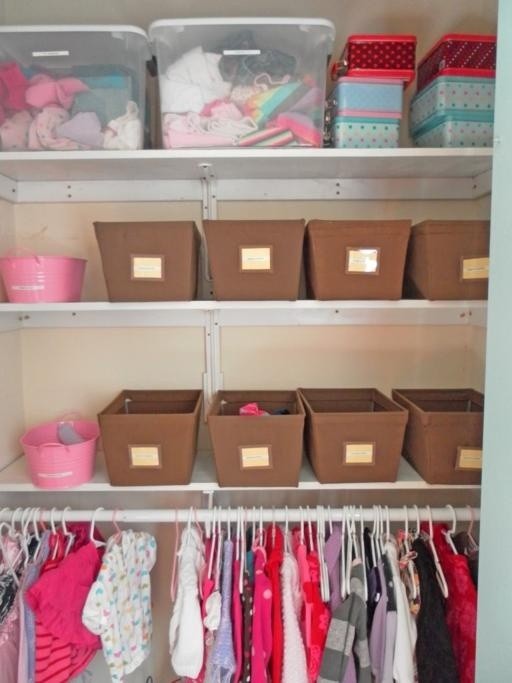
[1,506,483,614]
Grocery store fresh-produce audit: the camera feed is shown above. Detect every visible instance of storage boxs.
[208,391,306,487]
[332,118,401,148]
[307,220,412,300]
[395,389,484,485]
[332,77,403,117]
[407,220,489,300]
[411,114,495,148]
[300,388,409,485]
[149,17,335,147]
[411,76,495,136]
[202,219,305,302]
[90,222,201,300]
[418,34,498,90]
[98,388,204,486]
[334,35,417,90]
[0,25,148,151]
